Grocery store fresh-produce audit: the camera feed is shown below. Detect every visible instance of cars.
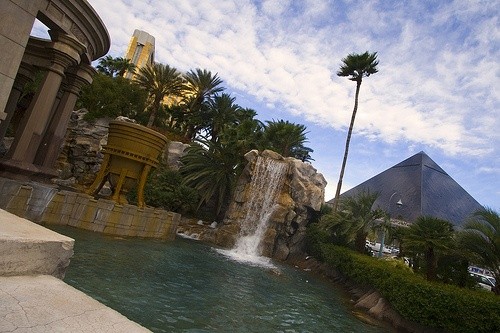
[364,239,415,267]
[467,266,497,286]
